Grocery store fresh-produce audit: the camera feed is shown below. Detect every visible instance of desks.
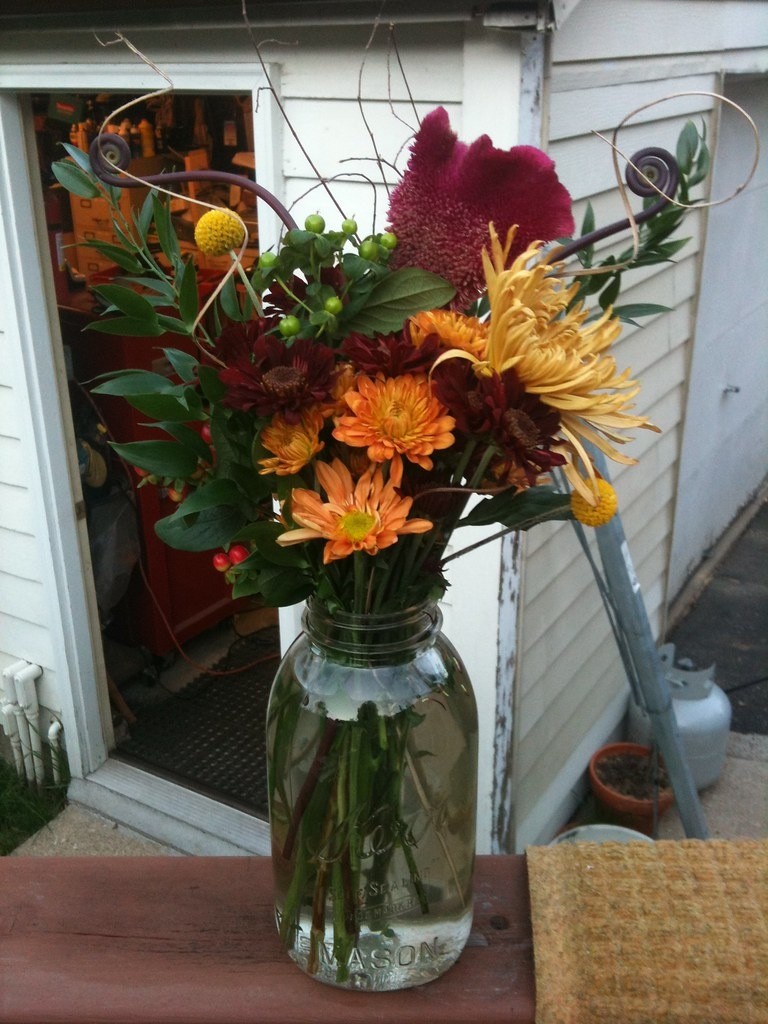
[0,858,539,1023]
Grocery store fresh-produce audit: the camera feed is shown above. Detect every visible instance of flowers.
[51,110,712,968]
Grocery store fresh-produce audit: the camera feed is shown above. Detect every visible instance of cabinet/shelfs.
[53,267,272,675]
[61,154,165,276]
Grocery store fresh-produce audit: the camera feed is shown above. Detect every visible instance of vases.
[265,586,479,995]
[589,740,675,839]
